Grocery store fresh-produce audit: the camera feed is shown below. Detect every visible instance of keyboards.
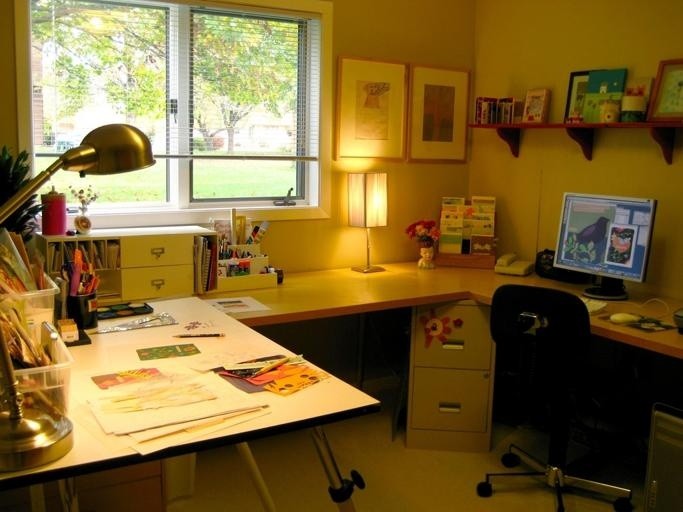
[575,296,607,315]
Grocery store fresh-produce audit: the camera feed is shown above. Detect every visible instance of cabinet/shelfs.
[77,460,167,512]
[464,121,683,165]
[35,225,220,306]
[409,299,496,451]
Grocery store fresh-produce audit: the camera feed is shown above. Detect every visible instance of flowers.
[69,184,99,215]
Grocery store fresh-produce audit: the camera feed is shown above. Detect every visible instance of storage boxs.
[0,321,74,417]
[0,266,61,342]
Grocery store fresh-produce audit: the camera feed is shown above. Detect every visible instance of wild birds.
[574,216,610,245]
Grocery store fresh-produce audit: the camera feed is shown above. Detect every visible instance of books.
[438,196,496,256]
[194,236,217,295]
[86,341,334,458]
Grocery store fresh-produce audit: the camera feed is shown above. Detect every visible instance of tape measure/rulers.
[222,354,304,370]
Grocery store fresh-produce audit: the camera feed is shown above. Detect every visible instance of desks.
[0,258,383,512]
[74,261,682,512]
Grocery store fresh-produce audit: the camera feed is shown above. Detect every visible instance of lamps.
[347,172,389,273]
[0,125,157,474]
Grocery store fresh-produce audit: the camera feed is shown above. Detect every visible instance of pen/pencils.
[172,333,224,338]
[252,358,289,377]
[61,249,101,296]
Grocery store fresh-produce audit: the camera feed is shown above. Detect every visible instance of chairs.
[475,283,637,512]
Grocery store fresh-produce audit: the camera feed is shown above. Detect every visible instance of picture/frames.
[645,58,683,122]
[406,62,471,164]
[333,53,408,162]
[564,69,604,124]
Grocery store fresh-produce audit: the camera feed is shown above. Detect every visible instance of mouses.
[610,312,642,324]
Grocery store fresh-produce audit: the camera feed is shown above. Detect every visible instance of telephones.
[494,253,535,277]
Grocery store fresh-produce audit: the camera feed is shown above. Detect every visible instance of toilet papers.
[40,193,67,236]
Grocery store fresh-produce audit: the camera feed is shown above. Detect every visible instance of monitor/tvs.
[551,192,657,300]
